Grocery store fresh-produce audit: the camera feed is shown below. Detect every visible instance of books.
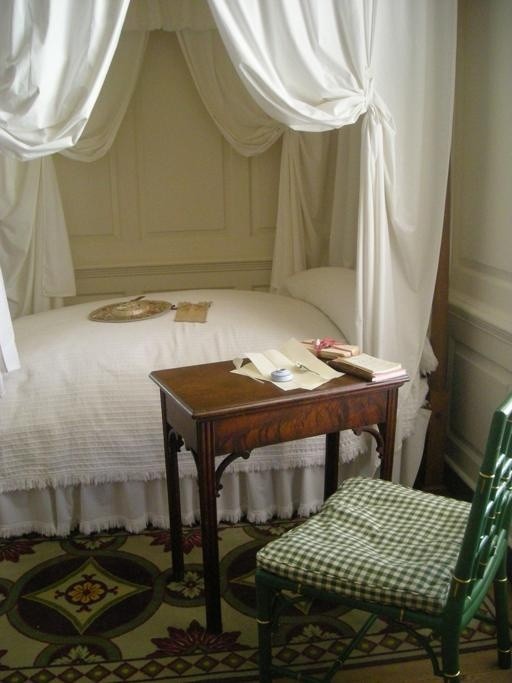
[326,348,407,383]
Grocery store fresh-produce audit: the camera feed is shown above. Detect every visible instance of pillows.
[285,264,438,379]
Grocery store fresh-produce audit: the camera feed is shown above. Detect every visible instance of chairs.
[255,391,511,683]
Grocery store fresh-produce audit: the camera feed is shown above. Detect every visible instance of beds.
[1,0,460,538]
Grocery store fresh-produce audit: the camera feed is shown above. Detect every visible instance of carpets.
[1,514,499,683]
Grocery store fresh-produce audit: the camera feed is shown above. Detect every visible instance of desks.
[149,358,409,635]
